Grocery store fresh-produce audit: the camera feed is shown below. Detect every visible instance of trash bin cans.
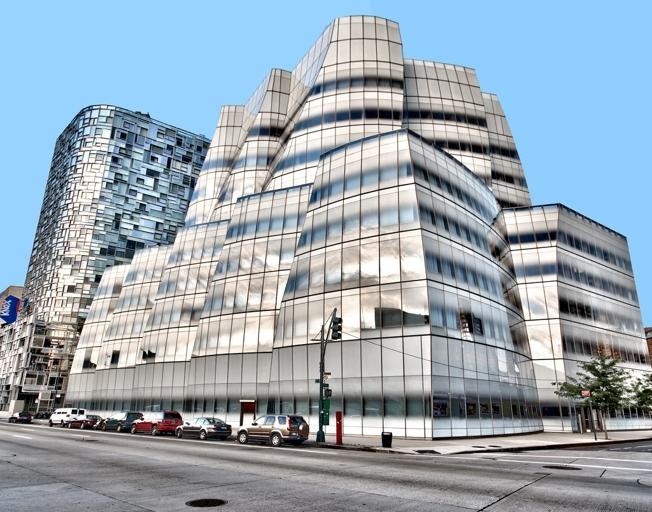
[382,432,392,447]
